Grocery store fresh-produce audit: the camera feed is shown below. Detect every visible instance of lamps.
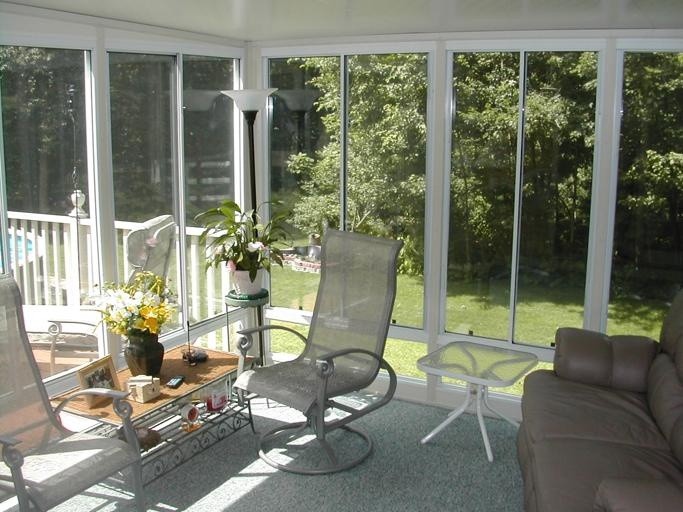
[223,86,274,238]
[276,86,320,176]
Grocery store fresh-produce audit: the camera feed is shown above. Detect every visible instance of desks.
[223,290,271,408]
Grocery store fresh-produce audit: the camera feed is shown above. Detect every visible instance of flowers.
[87,268,174,339]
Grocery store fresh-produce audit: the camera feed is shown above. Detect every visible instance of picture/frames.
[72,352,121,407]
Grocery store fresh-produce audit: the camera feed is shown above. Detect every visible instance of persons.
[86,369,115,390]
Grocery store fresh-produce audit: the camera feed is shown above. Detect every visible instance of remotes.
[166,375,184,388]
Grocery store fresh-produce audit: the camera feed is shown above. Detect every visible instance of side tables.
[416,338,539,463]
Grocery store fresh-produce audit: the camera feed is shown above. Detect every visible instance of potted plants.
[196,195,292,296]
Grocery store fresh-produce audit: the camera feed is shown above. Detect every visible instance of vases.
[123,327,166,375]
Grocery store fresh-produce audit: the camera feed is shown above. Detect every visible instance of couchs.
[517,290,683,512]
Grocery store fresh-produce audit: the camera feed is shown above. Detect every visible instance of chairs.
[27,214,174,374]
[1,273,143,510]
[233,227,404,473]
